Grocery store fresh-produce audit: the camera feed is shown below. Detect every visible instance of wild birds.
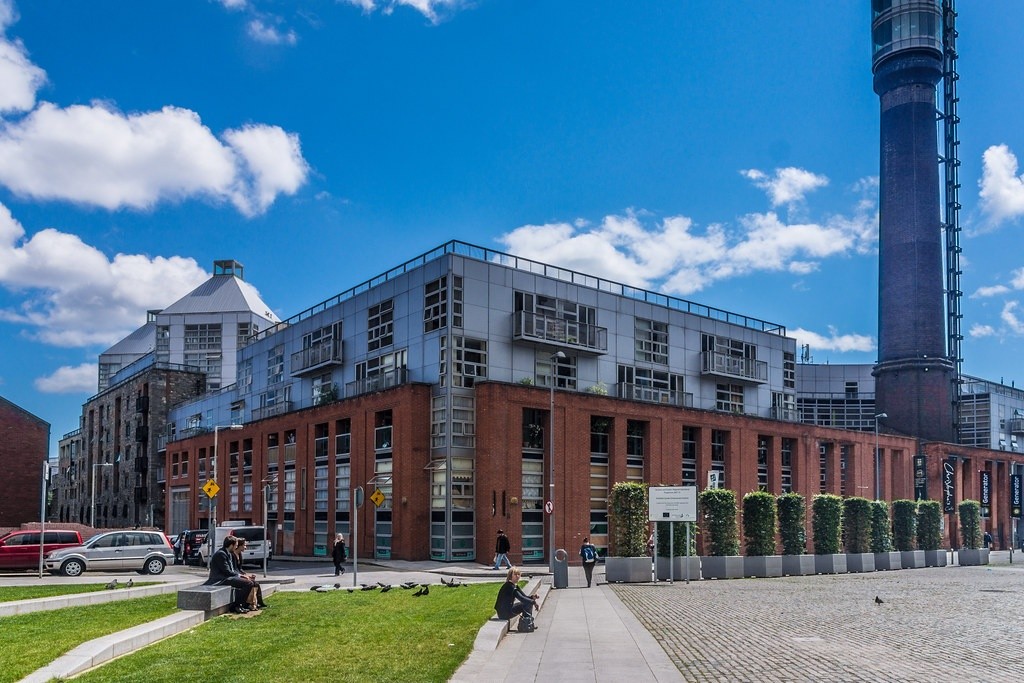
[875,596,884,606]
[310,577,467,597]
[104,578,118,590]
[126,579,134,590]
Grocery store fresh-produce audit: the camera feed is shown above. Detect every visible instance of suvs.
[44,528,174,577]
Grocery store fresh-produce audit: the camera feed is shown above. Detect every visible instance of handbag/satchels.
[511,603,535,632]
[247,586,259,610]
[493,553,498,563]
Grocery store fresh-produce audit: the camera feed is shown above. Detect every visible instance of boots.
[257,591,267,607]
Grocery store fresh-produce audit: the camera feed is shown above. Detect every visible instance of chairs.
[125,537,128,545]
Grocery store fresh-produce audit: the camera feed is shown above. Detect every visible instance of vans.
[197,525,273,568]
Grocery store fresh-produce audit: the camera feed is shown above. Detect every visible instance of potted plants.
[871,499,902,569]
[893,498,926,569]
[652,484,701,580]
[696,488,745,579]
[811,493,846,574]
[917,501,948,567]
[603,479,651,582]
[843,497,880,572]
[741,490,782,577]
[957,498,990,565]
[775,491,816,575]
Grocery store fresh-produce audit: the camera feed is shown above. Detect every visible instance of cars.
[0,530,83,569]
[168,529,206,566]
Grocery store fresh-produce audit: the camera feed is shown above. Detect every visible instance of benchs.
[177,584,236,610]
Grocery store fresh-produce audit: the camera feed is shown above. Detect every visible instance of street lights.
[207,424,245,573]
[874,412,888,501]
[92,461,114,529]
[549,349,566,572]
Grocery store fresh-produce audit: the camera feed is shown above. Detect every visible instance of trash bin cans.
[554,549,568,589]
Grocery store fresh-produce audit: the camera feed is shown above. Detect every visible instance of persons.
[579,536,599,588]
[494,528,513,569]
[287,433,295,444]
[1021,539,1024,553]
[201,536,268,614]
[984,531,992,549]
[493,567,541,633]
[330,531,348,576]
[171,533,184,565]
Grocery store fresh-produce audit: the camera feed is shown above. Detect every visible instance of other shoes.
[493,568,499,570]
[506,568,509,569]
[334,574,339,576]
[534,627,538,630]
[233,606,250,613]
[341,569,345,574]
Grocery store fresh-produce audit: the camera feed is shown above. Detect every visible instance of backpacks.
[582,544,595,563]
